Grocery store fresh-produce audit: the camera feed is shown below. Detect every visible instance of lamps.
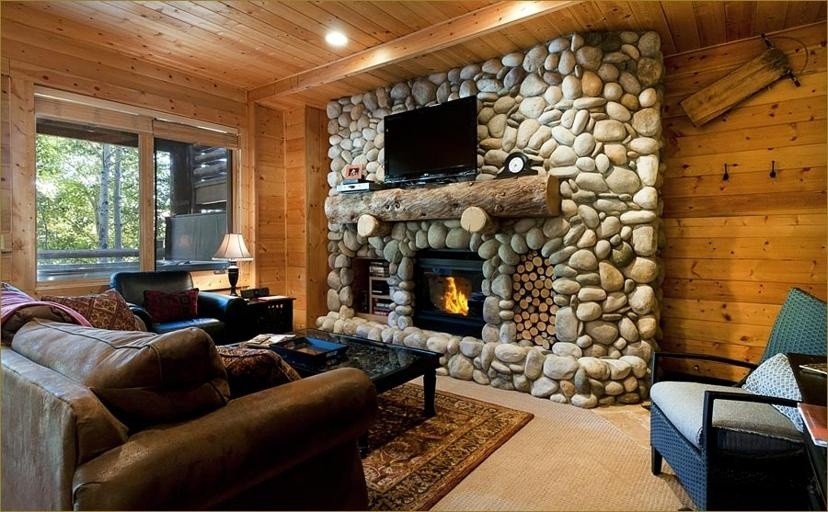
[213,234,251,294]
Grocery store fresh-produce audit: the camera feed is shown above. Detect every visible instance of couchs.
[1,281,375,512]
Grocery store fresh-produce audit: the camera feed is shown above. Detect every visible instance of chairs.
[649,288,827,510]
[110,270,246,344]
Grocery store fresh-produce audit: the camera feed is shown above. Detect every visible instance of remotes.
[253,336,271,345]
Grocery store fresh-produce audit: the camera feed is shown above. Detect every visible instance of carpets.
[356,381,535,511]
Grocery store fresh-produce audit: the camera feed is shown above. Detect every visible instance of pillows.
[217,348,301,398]
[743,353,805,432]
[143,288,200,322]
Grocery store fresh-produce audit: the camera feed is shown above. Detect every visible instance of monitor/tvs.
[383,95,478,189]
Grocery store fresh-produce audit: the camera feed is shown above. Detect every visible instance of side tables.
[216,288,296,333]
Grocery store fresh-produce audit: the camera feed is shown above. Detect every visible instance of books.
[257,296,289,301]
[797,402,828,447]
[799,363,827,375]
[369,262,392,317]
[245,333,297,349]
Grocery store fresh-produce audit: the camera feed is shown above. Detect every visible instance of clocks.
[496,151,538,178]
